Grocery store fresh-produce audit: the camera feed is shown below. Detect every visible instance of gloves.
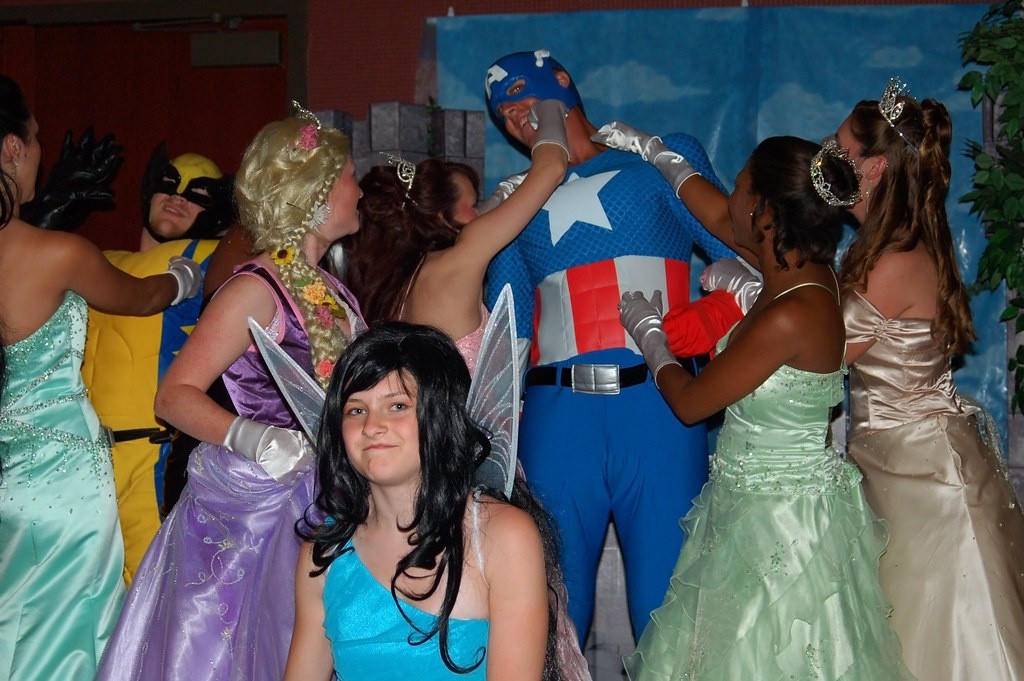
[662,288,744,356]
[527,97,571,158]
[701,256,763,315]
[477,169,528,215]
[22,124,126,230]
[225,416,316,486]
[591,120,701,198]
[616,289,683,391]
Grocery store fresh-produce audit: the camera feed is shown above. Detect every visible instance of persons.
[91,97,376,681]
[346,100,598,679]
[1,70,212,679]
[280,320,594,680]
[471,48,743,667]
[698,77,1023,680]
[593,120,918,681]
[19,116,240,591]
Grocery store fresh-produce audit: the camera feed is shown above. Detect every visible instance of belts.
[521,355,713,396]
